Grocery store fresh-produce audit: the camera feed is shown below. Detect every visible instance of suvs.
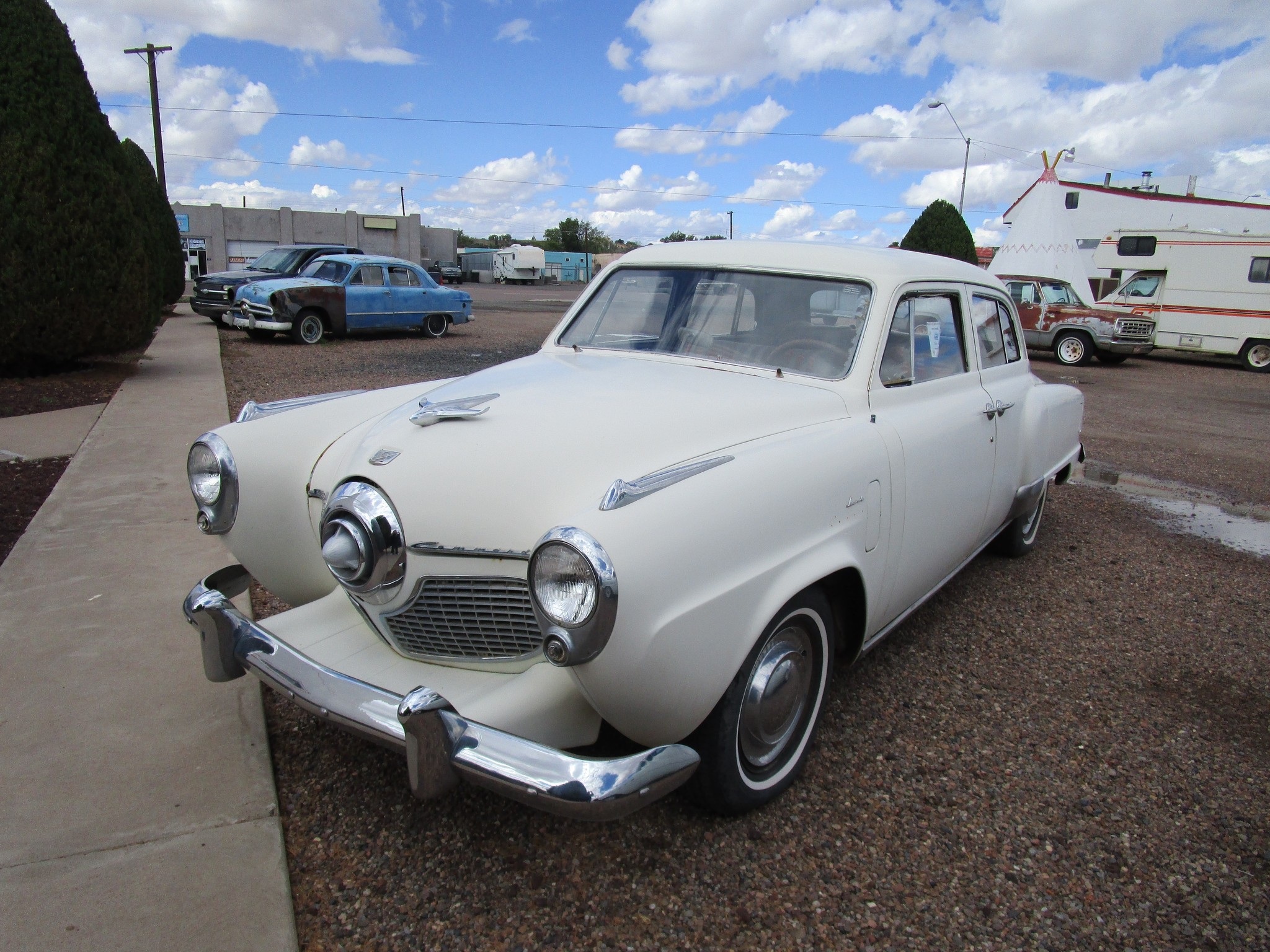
[189,244,367,329]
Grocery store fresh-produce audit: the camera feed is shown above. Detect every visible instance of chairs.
[777,320,857,381]
[687,332,772,369]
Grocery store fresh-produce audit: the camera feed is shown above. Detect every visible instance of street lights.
[1241,193,1261,203]
[927,102,971,214]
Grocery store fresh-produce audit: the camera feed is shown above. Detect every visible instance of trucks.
[1094,229,1270,370]
[493,244,546,285]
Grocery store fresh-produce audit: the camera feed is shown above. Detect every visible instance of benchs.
[784,321,960,384]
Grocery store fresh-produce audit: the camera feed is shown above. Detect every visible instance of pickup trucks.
[427,260,463,284]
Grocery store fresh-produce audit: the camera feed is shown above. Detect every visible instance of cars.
[221,254,475,337]
[995,274,1158,366]
[184,240,1087,823]
